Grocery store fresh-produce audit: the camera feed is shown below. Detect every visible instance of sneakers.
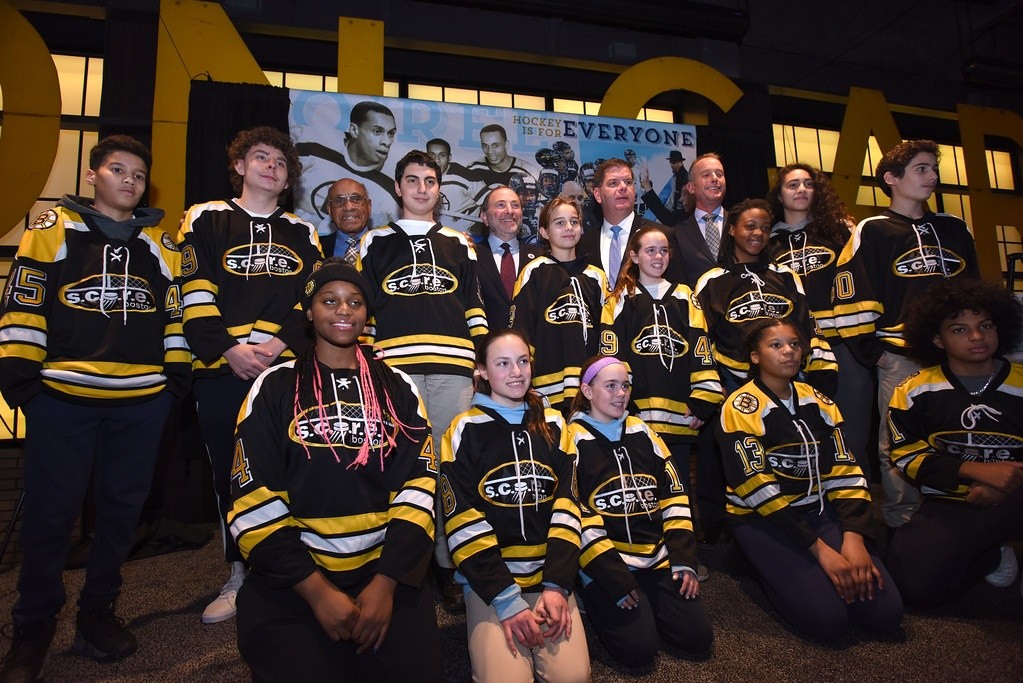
[72,600,137,660]
[0,623,56,683]
[202,573,244,624]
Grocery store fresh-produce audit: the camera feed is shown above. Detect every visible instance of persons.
[479,186,545,334]
[319,179,375,266]
[569,357,713,676]
[438,331,592,683]
[885,279,1023,612]
[229,262,440,683]
[359,150,492,611]
[671,154,733,289]
[717,319,904,641]
[832,142,980,526]
[581,161,677,293]
[290,101,693,238]
[767,164,874,489]
[596,225,722,580]
[0,135,190,683]
[177,129,322,621]
[503,192,610,415]
[693,204,839,546]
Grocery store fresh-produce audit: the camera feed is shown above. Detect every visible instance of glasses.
[330,195,366,208]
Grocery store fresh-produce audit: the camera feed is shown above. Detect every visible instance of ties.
[499,241,517,301]
[702,213,720,262]
[343,236,361,267]
[608,226,623,291]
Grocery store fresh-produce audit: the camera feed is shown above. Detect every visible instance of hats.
[304,257,367,308]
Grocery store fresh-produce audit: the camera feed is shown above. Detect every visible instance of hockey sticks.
[439,208,483,223]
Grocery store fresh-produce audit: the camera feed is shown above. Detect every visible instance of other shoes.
[439,569,465,614]
[697,560,709,582]
